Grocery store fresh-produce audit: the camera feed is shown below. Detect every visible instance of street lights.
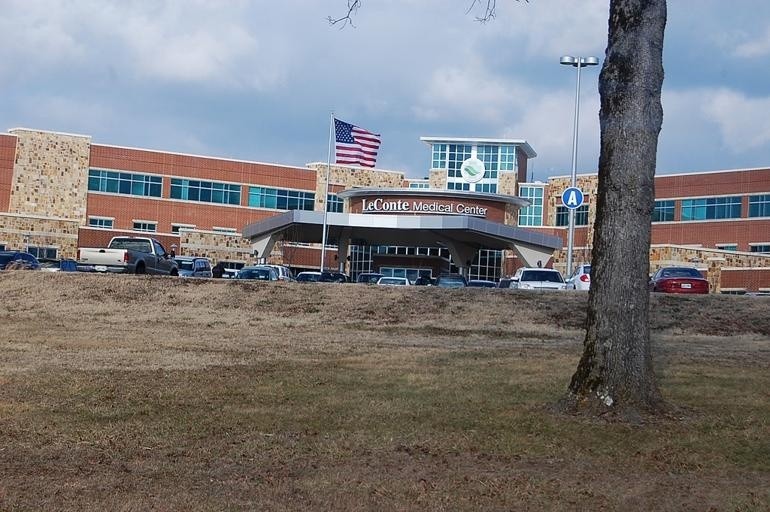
[559,54,599,294]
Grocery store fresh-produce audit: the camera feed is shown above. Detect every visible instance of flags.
[333,117,380,169]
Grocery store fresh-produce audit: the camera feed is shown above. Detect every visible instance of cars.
[434,273,467,289]
[566,263,592,292]
[376,276,411,286]
[36,257,65,272]
[173,258,322,284]
[0,250,41,270]
[466,280,498,288]
[355,273,386,284]
[648,266,711,295]
[510,267,567,292]
[319,271,349,283]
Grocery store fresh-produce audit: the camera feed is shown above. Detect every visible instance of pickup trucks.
[75,235,178,277]
